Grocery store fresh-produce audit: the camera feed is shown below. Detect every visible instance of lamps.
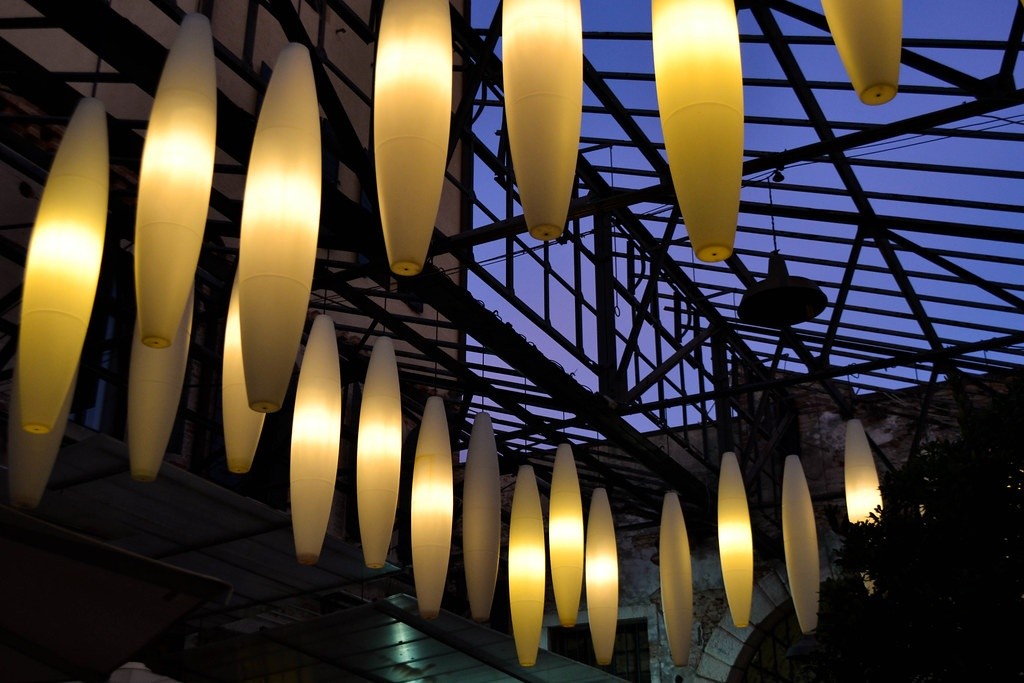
[5,55,693,671]
[134,1,218,351]
[781,454,821,635]
[717,451,754,629]
[501,0,584,241]
[373,0,452,277]
[820,0,903,107]
[237,0,321,414]
[841,406,884,597]
[650,0,745,262]
[737,181,829,328]
